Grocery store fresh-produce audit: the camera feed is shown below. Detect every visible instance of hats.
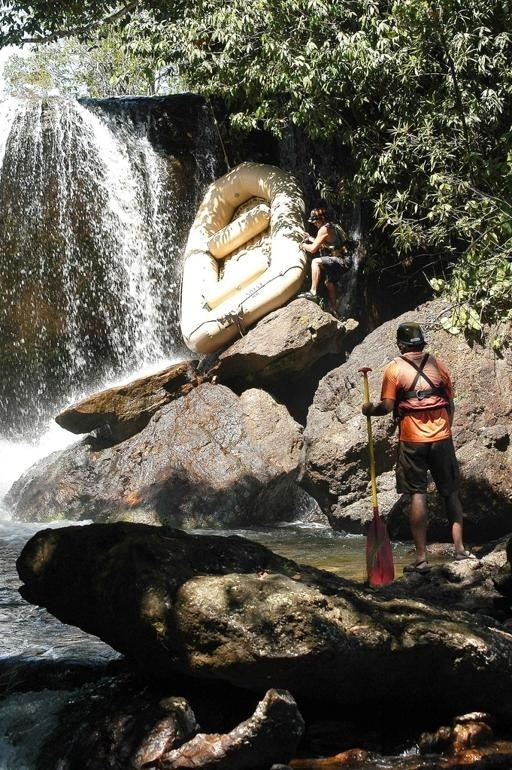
[308,208,328,223]
[396,322,427,345]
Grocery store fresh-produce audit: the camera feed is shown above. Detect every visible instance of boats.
[177,156,312,352]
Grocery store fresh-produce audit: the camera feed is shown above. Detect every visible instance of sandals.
[454,549,477,560]
[403,559,431,574]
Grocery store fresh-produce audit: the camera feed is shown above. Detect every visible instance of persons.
[295,206,356,319]
[361,320,474,573]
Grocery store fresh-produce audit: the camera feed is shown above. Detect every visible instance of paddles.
[354,366,394,584]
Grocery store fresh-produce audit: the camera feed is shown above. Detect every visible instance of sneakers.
[296,291,319,303]
